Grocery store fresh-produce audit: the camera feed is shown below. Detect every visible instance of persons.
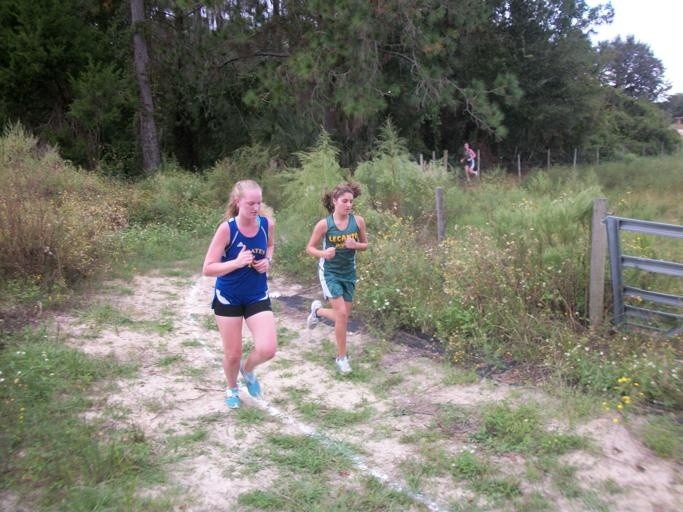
[459,141,478,182]
[306,182,369,376]
[202,178,278,412]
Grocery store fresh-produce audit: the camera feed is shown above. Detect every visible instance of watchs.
[264,256,273,267]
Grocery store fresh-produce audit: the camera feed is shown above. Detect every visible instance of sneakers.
[307,299,322,330]
[225,388,241,410]
[240,364,260,397]
[335,356,353,376]
